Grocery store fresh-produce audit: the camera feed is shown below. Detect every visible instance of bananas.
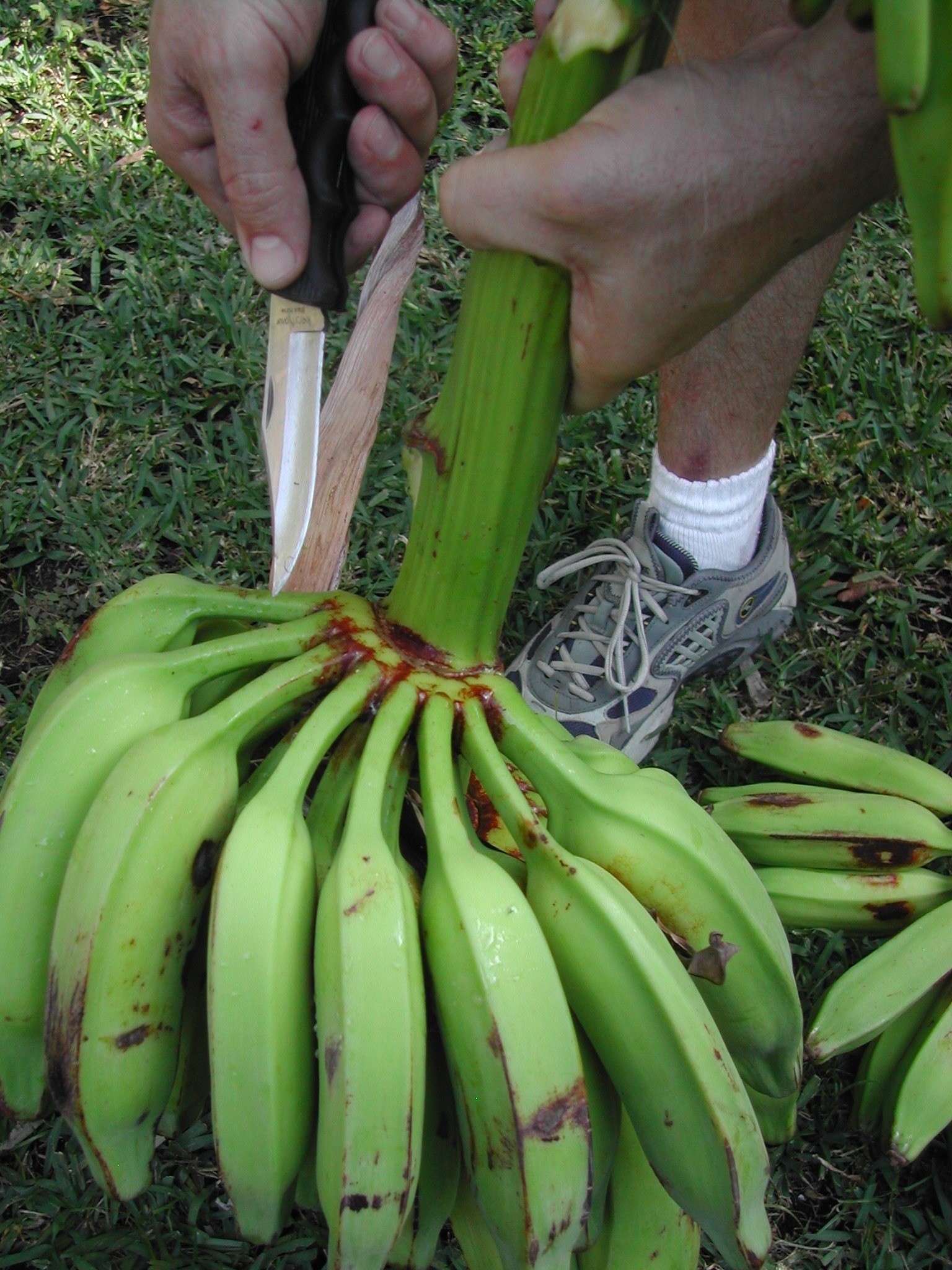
[0,572,808,1270]
[685,719,952,1171]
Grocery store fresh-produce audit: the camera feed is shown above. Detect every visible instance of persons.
[146,1,899,765]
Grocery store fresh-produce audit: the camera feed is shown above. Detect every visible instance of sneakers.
[506,497,798,767]
